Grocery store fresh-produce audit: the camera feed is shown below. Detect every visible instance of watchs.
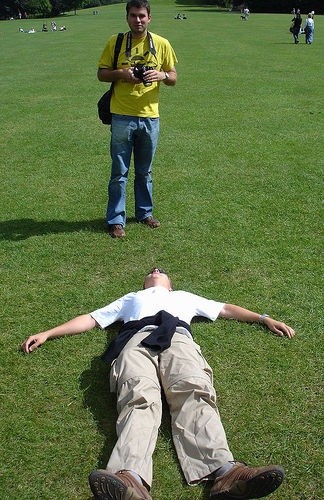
[165,72,169,80]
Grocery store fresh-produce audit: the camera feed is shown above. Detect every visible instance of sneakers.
[208,461,284,500]
[89,469,152,500]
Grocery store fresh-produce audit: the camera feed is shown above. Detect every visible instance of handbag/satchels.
[97,89,112,124]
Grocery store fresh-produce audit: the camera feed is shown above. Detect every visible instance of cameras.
[133,64,154,87]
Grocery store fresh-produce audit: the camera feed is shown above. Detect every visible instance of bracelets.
[260,314,268,324]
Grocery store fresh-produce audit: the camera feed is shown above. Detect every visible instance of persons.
[175,13,188,19]
[304,13,314,45]
[18,20,67,34]
[241,8,250,20]
[21,268,295,500]
[289,13,302,44]
[97,0,178,238]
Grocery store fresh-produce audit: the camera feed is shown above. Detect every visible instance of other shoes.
[142,216,160,227]
[109,224,125,238]
